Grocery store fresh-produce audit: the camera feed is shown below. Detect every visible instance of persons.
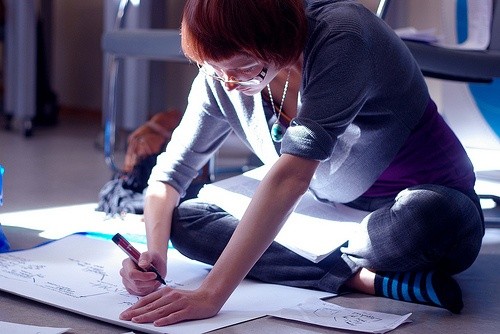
[119,0,486,327]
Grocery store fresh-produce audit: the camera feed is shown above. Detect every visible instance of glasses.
[198,57,272,87]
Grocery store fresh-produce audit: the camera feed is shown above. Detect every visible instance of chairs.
[373,0,500,207]
[101,0,199,174]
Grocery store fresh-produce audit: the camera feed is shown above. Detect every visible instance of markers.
[112,233,169,287]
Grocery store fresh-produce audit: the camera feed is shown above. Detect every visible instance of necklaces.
[266,66,291,143]
